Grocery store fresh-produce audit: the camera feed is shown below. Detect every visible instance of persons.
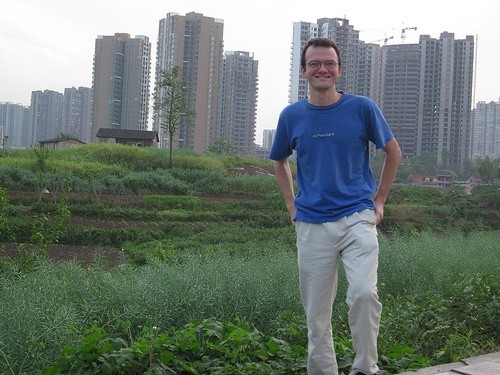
[269,36,404,375]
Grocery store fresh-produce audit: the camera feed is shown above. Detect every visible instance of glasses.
[303,60,339,69]
